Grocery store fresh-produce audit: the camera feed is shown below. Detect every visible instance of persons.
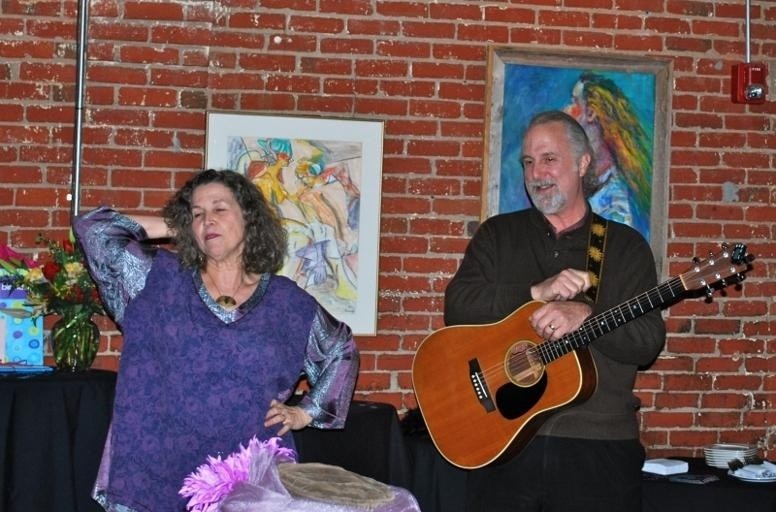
[72,168,359,512]
[443,110,667,511]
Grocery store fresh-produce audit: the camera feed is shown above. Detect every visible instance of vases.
[47,306,102,375]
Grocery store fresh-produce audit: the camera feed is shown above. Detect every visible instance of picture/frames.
[204,105,385,340]
[479,43,675,322]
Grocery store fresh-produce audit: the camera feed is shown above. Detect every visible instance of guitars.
[411,242,749,470]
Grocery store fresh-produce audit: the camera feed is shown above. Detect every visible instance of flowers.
[7,246,114,354]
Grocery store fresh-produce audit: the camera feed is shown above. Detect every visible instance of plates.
[703,443,775,483]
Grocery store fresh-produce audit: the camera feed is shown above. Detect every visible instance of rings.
[548,324,555,331]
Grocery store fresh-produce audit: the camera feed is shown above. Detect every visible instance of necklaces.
[206,267,245,308]
